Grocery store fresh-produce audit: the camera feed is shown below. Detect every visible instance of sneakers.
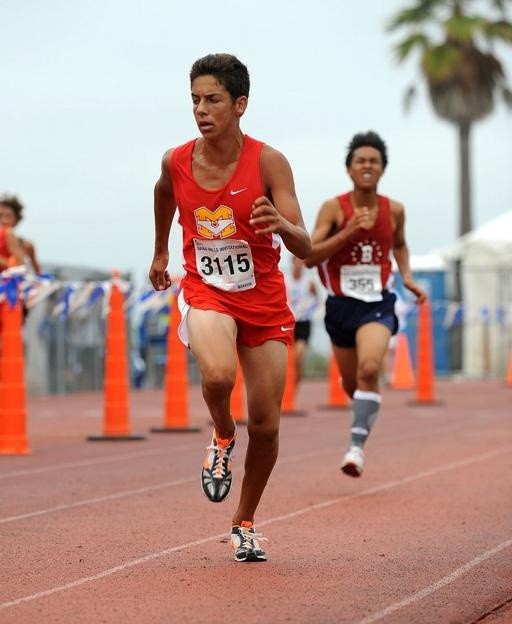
[231,520,267,562]
[340,445,365,479]
[200,415,237,504]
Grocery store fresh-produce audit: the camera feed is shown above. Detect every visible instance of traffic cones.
[0,254,33,457]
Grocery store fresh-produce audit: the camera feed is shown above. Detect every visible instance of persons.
[305,132,428,478]
[149,53,313,562]
[0,193,41,325]
[284,256,320,382]
